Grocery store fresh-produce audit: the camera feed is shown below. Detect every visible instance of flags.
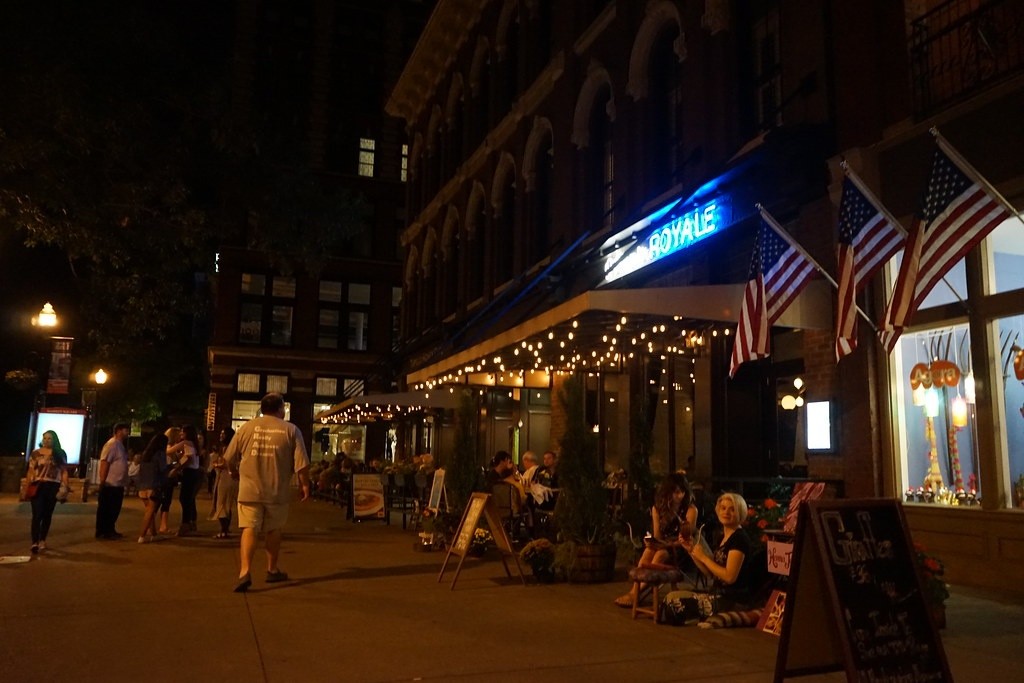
[728,219,816,378]
[833,174,907,365]
[880,141,1013,352]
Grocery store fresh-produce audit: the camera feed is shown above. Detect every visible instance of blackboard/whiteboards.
[449,492,516,558]
[428,468,448,512]
[776,498,953,683]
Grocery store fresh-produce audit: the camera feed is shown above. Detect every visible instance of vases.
[471,542,486,556]
[531,561,549,581]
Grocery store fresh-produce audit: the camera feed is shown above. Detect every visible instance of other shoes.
[95,531,124,540]
[233,574,252,593]
[175,525,198,537]
[39,540,46,550]
[160,527,176,534]
[139,537,146,544]
[151,535,166,542]
[265,569,289,582]
[30,544,40,554]
[213,532,226,539]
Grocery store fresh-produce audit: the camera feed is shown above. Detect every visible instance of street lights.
[19,300,57,499]
[93,368,108,459]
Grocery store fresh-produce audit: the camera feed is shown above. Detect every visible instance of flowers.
[747,498,787,531]
[913,539,946,584]
[471,527,494,545]
[519,538,557,564]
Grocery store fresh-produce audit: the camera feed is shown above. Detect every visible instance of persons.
[207,448,219,498]
[207,427,234,538]
[26,431,68,554]
[128,454,141,477]
[660,492,750,624]
[519,452,557,508]
[615,475,698,607]
[95,422,129,540]
[136,425,207,544]
[309,452,433,521]
[223,395,310,592]
[485,450,522,543]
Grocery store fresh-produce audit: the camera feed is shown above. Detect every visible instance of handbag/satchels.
[20,478,38,502]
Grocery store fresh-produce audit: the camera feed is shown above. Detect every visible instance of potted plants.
[553,373,621,582]
[440,385,485,553]
[626,567,681,624]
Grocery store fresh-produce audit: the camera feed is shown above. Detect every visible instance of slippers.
[615,593,634,608]
